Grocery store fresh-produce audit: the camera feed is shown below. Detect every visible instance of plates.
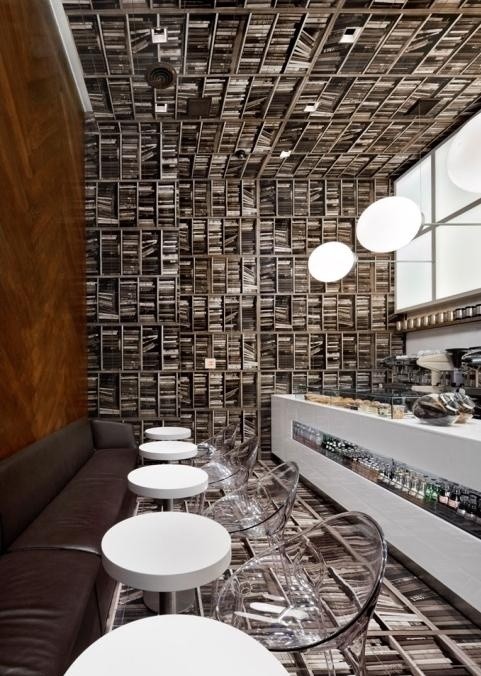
[457,412,473,423]
[424,414,458,426]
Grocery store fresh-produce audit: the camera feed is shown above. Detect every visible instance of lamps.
[306,241,393,284]
[359,195,481,255]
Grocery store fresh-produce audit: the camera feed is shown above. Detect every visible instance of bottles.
[294,424,481,526]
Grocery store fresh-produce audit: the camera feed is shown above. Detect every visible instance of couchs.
[1,415,141,676]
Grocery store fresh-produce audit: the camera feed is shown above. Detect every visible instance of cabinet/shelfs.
[271,392,481,631]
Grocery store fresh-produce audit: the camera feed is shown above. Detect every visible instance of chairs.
[207,462,300,581]
[197,423,240,465]
[202,436,260,494]
[216,512,389,676]
